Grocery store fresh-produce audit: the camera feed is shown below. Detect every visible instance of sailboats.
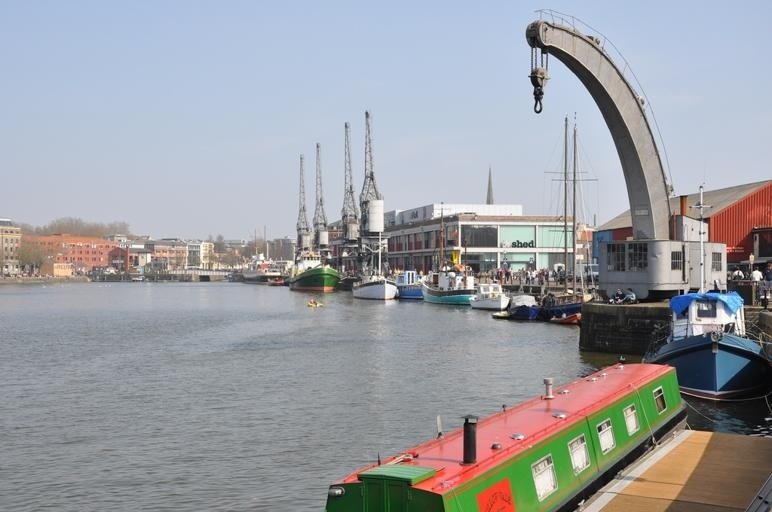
[540,111,595,322]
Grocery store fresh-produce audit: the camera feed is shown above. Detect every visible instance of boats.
[469,283,511,311]
[641,291,772,404]
[325,364,691,512]
[508,295,540,321]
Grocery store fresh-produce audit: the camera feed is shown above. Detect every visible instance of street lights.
[462,238,468,289]
[749,253,754,281]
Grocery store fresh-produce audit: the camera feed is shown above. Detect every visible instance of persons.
[413,267,424,279]
[727,260,772,303]
[388,265,400,277]
[539,289,549,307]
[544,293,556,307]
[612,288,625,303]
[619,288,636,304]
[477,265,566,286]
[442,262,451,272]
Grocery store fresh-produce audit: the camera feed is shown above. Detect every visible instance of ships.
[228,253,340,293]
[351,265,478,306]
